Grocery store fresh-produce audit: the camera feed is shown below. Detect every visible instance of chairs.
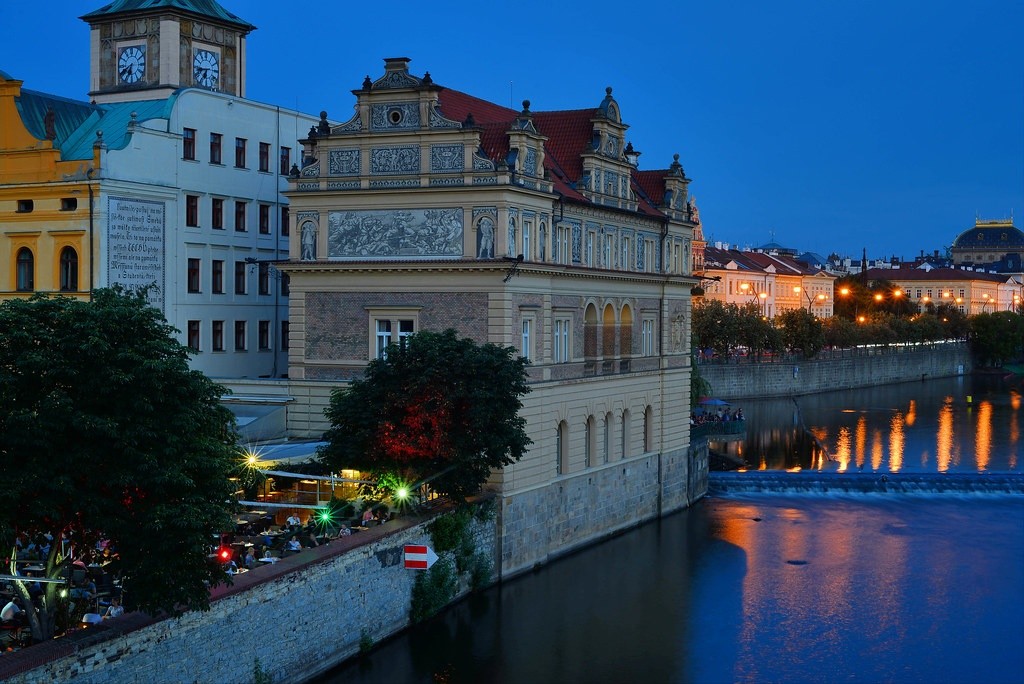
[231,511,378,566]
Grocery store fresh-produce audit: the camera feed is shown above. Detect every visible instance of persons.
[0,506,385,641]
[690,407,745,426]
[83,606,106,629]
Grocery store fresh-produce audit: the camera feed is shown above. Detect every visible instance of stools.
[0,626,17,645]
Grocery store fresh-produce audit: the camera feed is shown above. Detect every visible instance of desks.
[223,511,368,574]
[23,566,46,578]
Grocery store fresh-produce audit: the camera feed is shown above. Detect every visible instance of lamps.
[0,561,67,584]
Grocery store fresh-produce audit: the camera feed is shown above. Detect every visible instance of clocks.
[193,47,219,89]
[118,45,145,85]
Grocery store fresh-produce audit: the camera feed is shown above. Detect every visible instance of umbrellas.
[695,396,732,414]
[693,347,712,356]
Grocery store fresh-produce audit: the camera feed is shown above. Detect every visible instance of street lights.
[841,288,858,318]
[740,284,760,316]
[750,293,767,314]
[948,298,961,308]
[1008,295,1019,311]
[944,292,958,309]
[913,296,929,312]
[794,286,811,314]
[809,294,828,314]
[982,298,994,313]
[865,295,882,316]
